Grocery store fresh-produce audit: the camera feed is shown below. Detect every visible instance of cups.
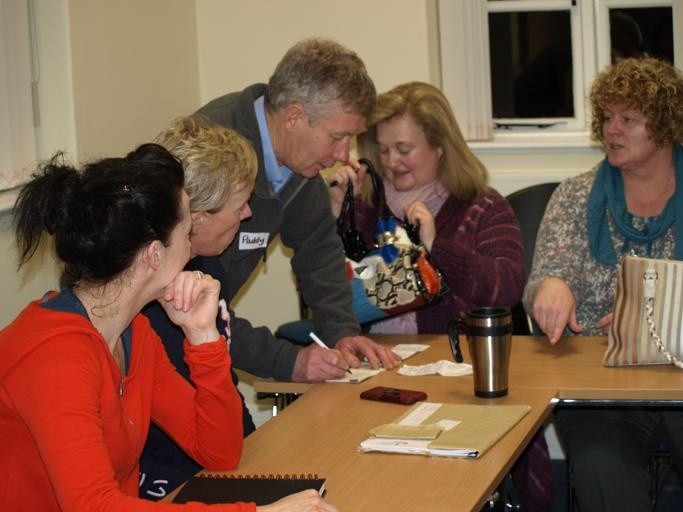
[447,305,515,398]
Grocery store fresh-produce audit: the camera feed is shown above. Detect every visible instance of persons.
[0,142,332,512]
[139,38,402,505]
[326,82,522,336]
[523,52,683,511]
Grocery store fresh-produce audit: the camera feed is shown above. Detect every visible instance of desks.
[254,335,682,419]
[152,393,562,511]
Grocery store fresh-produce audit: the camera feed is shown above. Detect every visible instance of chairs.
[503,182,569,512]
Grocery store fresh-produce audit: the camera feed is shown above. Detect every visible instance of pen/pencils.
[306,329,352,374]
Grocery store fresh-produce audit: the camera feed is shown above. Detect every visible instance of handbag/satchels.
[602,255,683,367]
[336,158,449,324]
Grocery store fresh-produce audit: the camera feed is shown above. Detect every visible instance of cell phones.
[360,386,426,406]
